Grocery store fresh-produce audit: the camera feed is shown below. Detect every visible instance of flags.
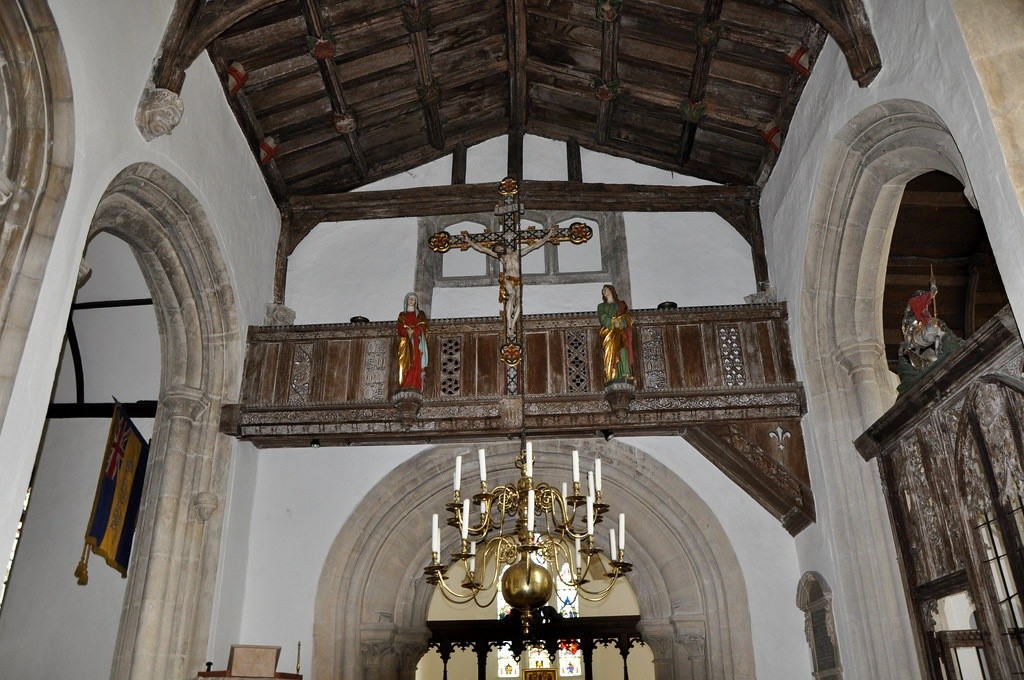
[85,403,148,578]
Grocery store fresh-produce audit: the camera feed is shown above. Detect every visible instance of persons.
[899,281,947,372]
[395,293,429,398]
[597,285,637,388]
[461,226,560,337]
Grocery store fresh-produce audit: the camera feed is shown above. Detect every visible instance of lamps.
[423,1,632,636]
